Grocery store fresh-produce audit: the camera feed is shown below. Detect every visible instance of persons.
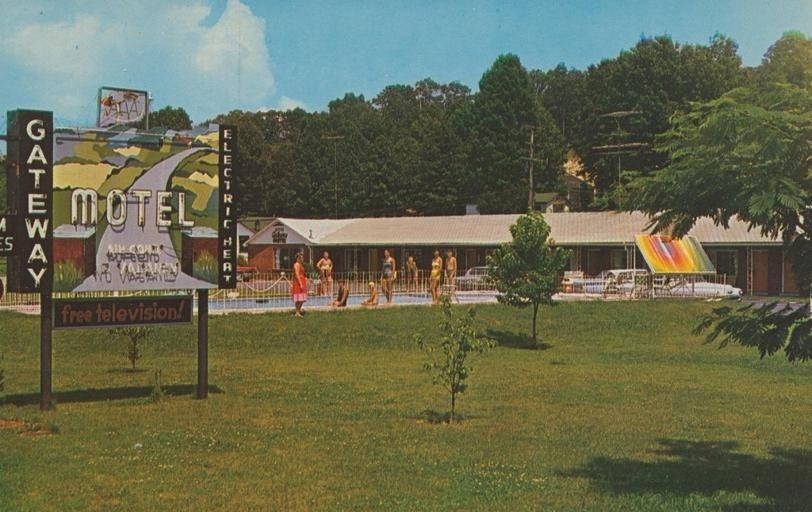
[317,251,333,295]
[444,249,459,304]
[292,252,308,318]
[381,248,395,304]
[328,279,349,306]
[406,255,418,293]
[430,248,443,305]
[361,282,379,306]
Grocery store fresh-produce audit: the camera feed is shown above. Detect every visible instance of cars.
[453,265,746,298]
